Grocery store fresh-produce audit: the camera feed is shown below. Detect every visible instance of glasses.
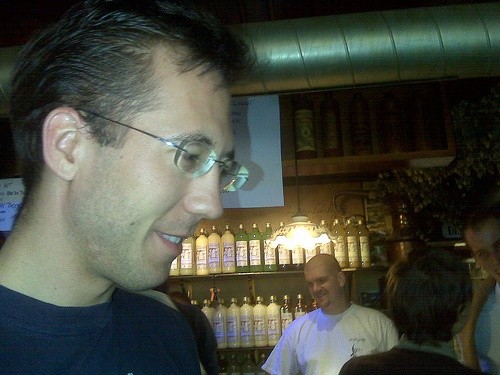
[74,106,248,192]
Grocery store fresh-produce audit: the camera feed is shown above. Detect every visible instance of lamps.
[266,94,339,250]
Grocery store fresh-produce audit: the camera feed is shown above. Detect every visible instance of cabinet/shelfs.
[168,82,455,351]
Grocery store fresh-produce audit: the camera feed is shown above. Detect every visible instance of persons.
[261,253,399,375]
[452,199,500,375]
[0,0,256,375]
[339,243,486,375]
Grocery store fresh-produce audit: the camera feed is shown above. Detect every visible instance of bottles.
[253,296,267,348]
[290,77,500,158]
[280,294,294,337]
[169,252,181,277]
[200,298,213,329]
[216,355,228,375]
[276,219,370,272]
[239,297,254,347]
[189,299,200,312]
[229,353,243,375]
[213,298,226,348]
[208,225,221,275]
[196,228,208,275]
[180,233,196,277]
[309,294,318,313]
[243,354,256,375]
[256,352,270,375]
[235,224,249,273]
[226,298,240,348]
[266,295,281,346]
[249,223,263,273]
[295,293,309,320]
[221,224,236,274]
[262,222,276,274]
